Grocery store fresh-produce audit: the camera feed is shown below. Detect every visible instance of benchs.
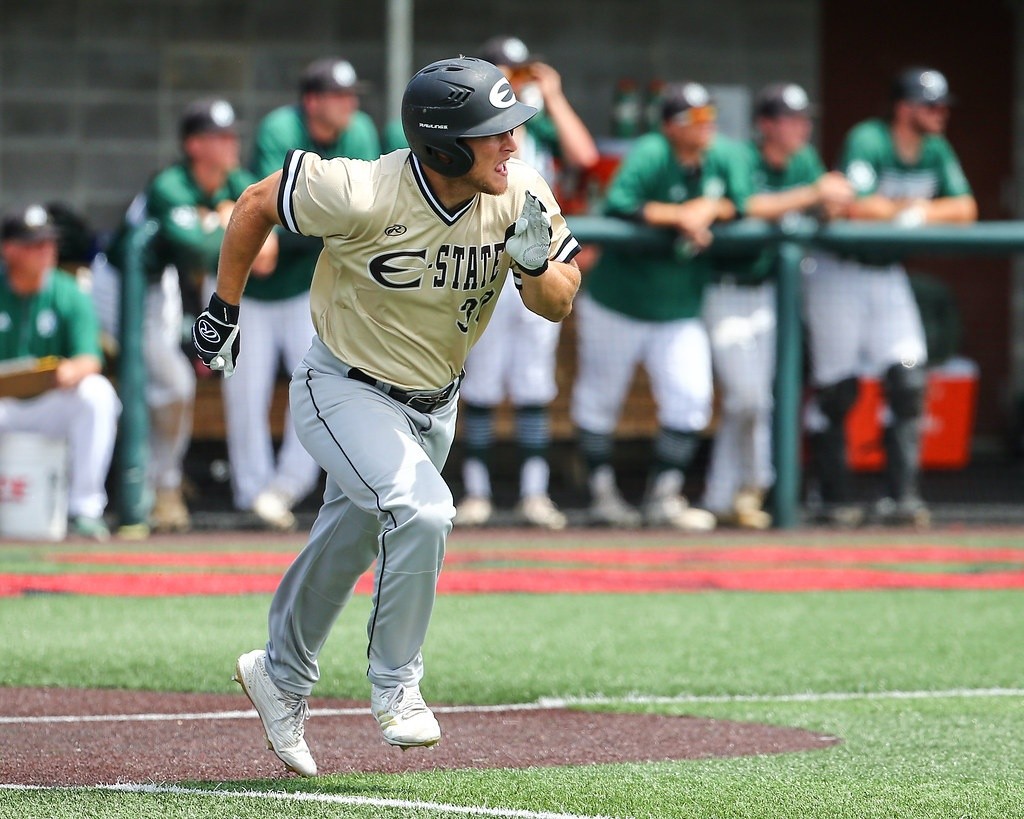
[184,357,715,446]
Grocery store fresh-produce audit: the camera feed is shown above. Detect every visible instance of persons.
[92,100,278,530]
[0,204,123,543]
[450,38,597,531]
[803,67,978,527]
[701,84,852,532]
[191,57,584,779]
[200,56,384,531]
[571,83,749,531]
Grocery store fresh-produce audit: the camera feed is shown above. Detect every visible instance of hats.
[660,82,712,125]
[471,35,541,67]
[301,58,369,92]
[901,70,949,105]
[180,98,250,136]
[752,81,814,118]
[0,204,63,241]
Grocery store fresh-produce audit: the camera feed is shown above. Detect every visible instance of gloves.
[190,294,238,380]
[505,191,551,278]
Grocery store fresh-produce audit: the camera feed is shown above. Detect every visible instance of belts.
[346,365,463,414]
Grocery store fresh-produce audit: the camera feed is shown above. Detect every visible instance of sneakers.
[233,649,316,779]
[370,680,440,746]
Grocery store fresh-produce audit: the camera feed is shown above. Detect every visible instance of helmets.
[401,56,538,177]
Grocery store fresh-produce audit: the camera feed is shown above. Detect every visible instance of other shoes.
[647,495,720,531]
[587,500,641,527]
[253,493,294,528]
[72,515,108,543]
[512,498,566,529]
[154,486,192,532]
[452,496,491,525]
[728,491,772,529]
[892,503,932,528]
